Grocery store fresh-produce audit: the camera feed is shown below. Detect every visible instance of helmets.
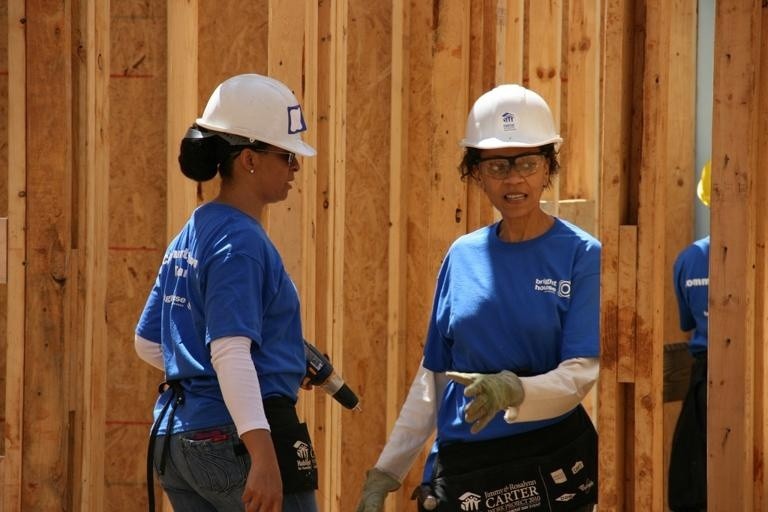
[697,160,711,208]
[464,84,561,150]
[196,74,317,157]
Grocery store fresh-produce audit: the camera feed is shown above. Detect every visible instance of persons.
[667,159,712,510]
[131,74,319,512]
[357,83,601,512]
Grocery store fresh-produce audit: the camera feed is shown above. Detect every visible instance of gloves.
[356,469,401,512]
[445,369,524,434]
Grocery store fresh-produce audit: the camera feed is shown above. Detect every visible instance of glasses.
[476,154,545,180]
[256,149,295,167]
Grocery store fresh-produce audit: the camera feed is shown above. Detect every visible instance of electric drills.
[303,338,363,413]
